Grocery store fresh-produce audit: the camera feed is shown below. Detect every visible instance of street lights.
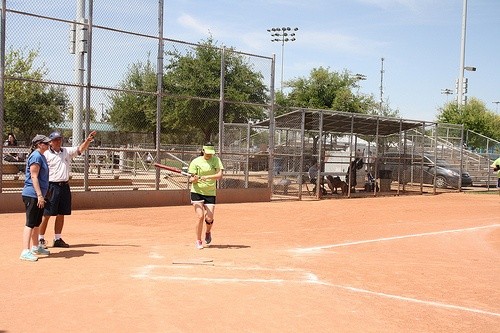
[266,24,299,91]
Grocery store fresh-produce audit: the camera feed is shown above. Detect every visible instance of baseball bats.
[154,164,204,180]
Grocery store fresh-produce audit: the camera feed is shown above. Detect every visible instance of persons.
[41,130,97,249]
[345,156,364,192]
[188,142,224,249]
[20,135,50,260]
[4,133,25,172]
[327,175,347,196]
[309,163,327,194]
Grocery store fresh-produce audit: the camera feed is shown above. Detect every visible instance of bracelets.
[37,194,42,197]
[87,138,92,141]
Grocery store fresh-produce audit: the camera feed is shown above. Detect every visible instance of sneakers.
[39,239,47,248]
[31,248,50,255]
[54,238,69,247]
[20,250,38,261]
[205,232,211,243]
[196,241,203,249]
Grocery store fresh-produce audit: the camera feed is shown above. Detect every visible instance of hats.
[203,146,215,154]
[32,135,52,146]
[50,132,62,140]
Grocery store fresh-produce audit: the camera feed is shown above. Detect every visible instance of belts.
[52,182,67,186]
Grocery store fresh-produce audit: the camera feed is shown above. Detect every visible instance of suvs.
[379,151,474,189]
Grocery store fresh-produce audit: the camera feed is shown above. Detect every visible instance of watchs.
[490,158,500,195]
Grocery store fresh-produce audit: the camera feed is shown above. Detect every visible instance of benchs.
[280,172,346,195]
